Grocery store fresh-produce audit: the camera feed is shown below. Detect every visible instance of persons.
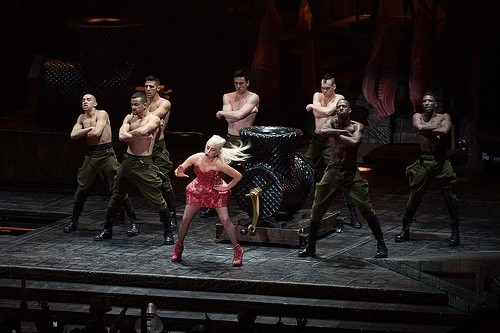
[144,77,177,231]
[94,93,175,245]
[216,71,259,148]
[63,94,141,235]
[172,135,244,266]
[305,74,362,229]
[394,93,460,246]
[298,100,388,258]
[0,295,307,333]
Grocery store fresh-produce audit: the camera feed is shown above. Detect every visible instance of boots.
[164,189,177,231]
[448,203,460,245]
[200,208,218,217]
[158,206,174,245]
[395,206,416,241]
[113,207,125,225]
[366,214,389,258]
[298,220,321,256]
[64,203,84,232]
[94,208,118,240]
[345,202,362,228]
[122,196,141,235]
[232,243,243,266]
[171,239,184,262]
